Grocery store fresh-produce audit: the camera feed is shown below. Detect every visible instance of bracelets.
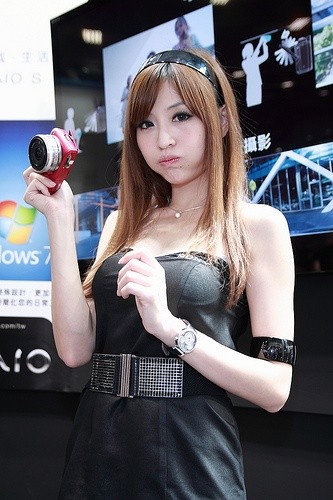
[249,335,298,366]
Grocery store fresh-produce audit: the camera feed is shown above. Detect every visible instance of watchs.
[161,317,197,357]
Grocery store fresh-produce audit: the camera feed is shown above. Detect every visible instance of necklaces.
[167,203,208,219]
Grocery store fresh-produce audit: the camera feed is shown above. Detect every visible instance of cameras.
[28,128,77,197]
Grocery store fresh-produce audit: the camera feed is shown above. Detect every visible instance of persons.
[121,74,132,133]
[21,46,300,500]
[171,16,204,50]
